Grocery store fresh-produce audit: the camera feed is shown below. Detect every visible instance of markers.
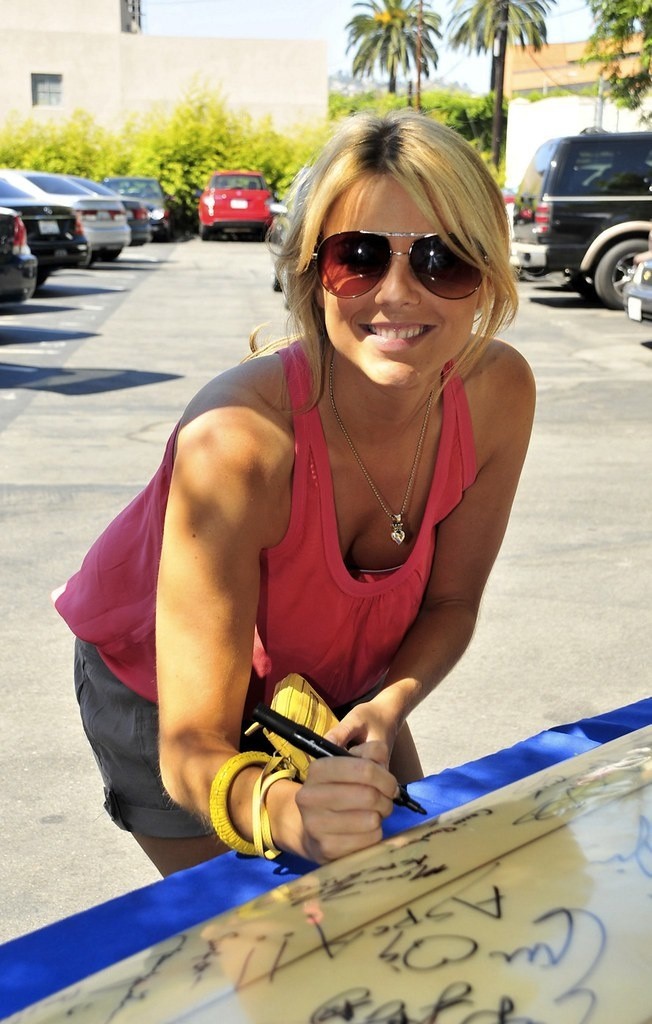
[251,702,428,816]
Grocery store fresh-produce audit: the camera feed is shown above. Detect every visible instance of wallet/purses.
[262,669,346,782]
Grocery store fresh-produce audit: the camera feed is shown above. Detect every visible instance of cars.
[0,170,153,262]
[102,177,174,243]
[198,169,274,242]
[0,175,90,290]
[0,208,39,305]
[621,259,652,326]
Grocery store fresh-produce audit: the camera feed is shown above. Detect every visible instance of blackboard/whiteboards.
[0,721,652,1024]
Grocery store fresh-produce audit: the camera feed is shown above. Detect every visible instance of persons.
[56,115,536,877]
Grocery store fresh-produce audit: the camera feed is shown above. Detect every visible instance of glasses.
[309,231,491,300]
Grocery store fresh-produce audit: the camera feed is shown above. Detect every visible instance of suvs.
[508,131,652,310]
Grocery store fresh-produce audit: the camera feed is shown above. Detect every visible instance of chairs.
[248,182,257,189]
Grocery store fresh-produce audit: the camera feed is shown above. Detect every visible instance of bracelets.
[209,751,280,855]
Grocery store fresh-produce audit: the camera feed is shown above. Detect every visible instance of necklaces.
[329,356,434,545]
